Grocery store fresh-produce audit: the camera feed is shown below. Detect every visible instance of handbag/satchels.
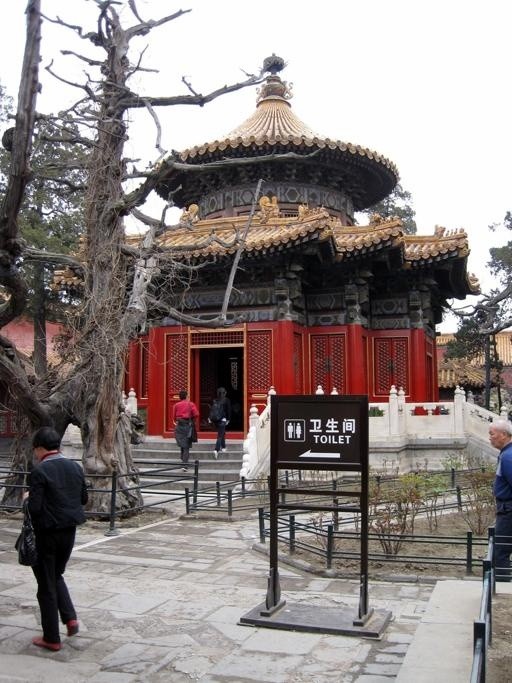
[186,418,197,442]
[14,499,39,566]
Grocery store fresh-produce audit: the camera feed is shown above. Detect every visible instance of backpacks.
[209,398,226,424]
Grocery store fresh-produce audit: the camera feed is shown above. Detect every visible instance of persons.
[22,425,90,654]
[207,387,233,459]
[171,389,200,473]
[487,419,512,581]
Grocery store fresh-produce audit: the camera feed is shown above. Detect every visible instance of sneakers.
[66,619,79,637]
[221,446,228,453]
[211,450,218,460]
[31,636,61,652]
